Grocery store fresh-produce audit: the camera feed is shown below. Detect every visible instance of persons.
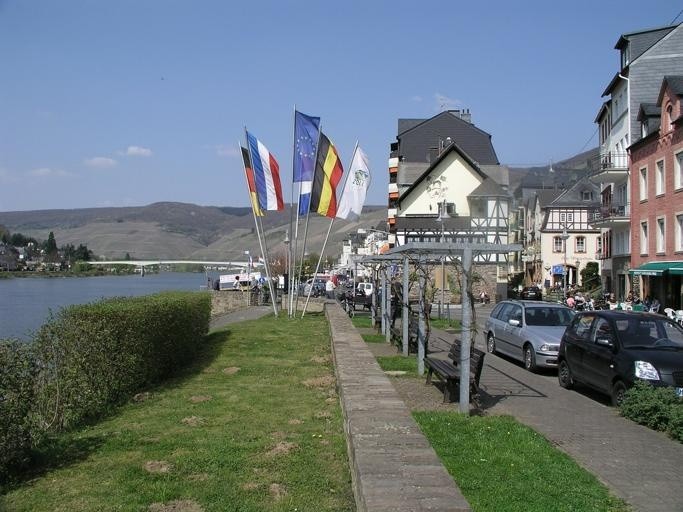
[480,291,487,303]
[250,275,258,292]
[555,278,661,313]
[259,276,265,284]
[531,279,542,289]
[214,279,220,291]
[233,276,241,291]
[325,278,336,299]
[391,277,402,303]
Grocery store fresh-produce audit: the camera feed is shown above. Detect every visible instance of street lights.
[281,226,290,310]
[559,227,570,303]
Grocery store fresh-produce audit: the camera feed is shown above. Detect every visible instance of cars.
[520,286,543,301]
[355,282,378,297]
[556,309,682,406]
[483,299,576,372]
[298,276,334,294]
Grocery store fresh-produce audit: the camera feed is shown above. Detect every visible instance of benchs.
[389,310,485,407]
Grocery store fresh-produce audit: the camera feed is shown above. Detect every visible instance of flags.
[241,112,372,223]
